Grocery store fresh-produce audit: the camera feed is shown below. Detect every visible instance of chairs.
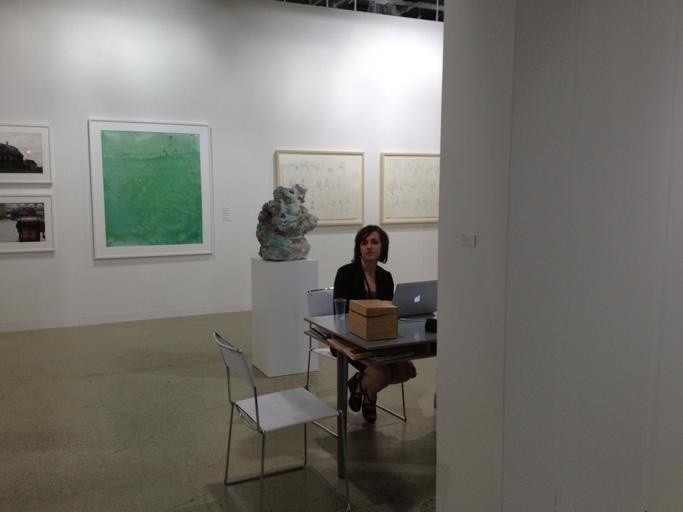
[304,286,408,439]
[215,334,338,487]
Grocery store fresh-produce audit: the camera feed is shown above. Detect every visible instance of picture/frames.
[88,120,211,260]
[274,148,440,228]
[1,123,54,254]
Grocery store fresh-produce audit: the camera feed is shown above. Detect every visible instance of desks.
[306,314,436,480]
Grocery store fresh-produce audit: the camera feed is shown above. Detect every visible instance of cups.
[334,298,347,320]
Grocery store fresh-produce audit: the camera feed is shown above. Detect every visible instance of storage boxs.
[344,298,398,340]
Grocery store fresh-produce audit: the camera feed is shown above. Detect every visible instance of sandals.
[361,387,377,423]
[347,372,363,412]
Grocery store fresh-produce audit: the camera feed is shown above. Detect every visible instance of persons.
[328,225,418,424]
[16,207,44,241]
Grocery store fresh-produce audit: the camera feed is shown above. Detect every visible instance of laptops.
[392,280,438,317]
